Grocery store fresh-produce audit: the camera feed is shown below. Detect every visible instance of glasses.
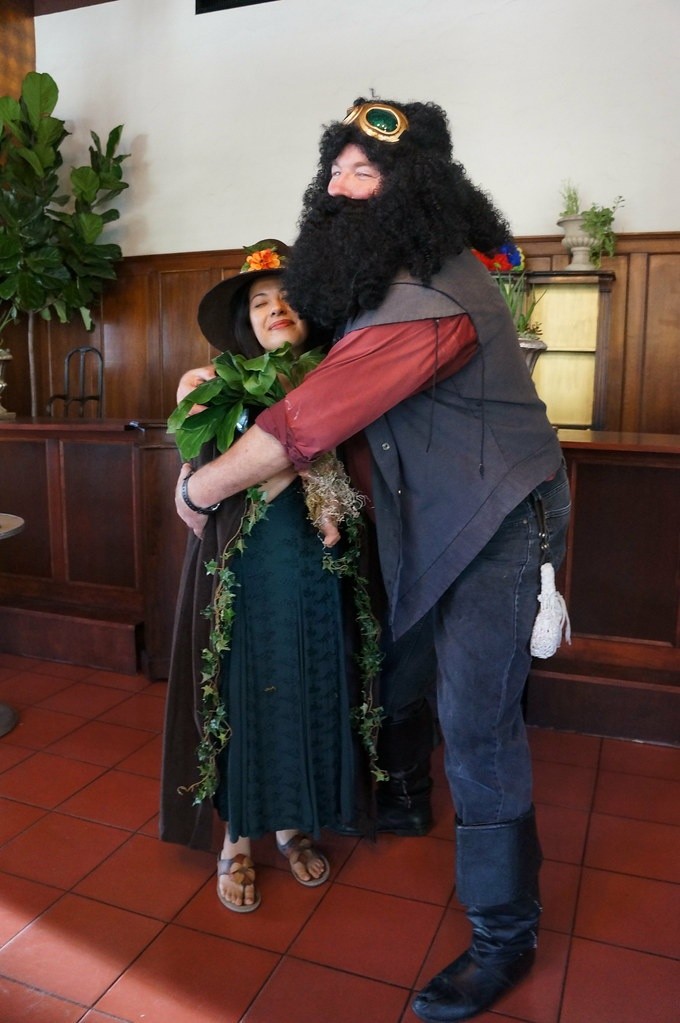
[340,103,411,144]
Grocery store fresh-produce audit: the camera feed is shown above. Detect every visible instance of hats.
[198,239,293,359]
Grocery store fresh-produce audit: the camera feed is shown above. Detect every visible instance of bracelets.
[179,476,221,515]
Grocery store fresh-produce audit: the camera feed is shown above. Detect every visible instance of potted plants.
[0,71,132,422]
[556,191,626,271]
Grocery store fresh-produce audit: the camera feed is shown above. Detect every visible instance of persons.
[149,239,437,909]
[173,95,572,1019]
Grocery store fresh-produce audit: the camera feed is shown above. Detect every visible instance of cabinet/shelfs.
[0,229,680,670]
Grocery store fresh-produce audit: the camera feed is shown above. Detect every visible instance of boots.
[409,803,543,1023]
[333,717,434,837]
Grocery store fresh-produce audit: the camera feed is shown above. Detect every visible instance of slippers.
[217,832,331,913]
